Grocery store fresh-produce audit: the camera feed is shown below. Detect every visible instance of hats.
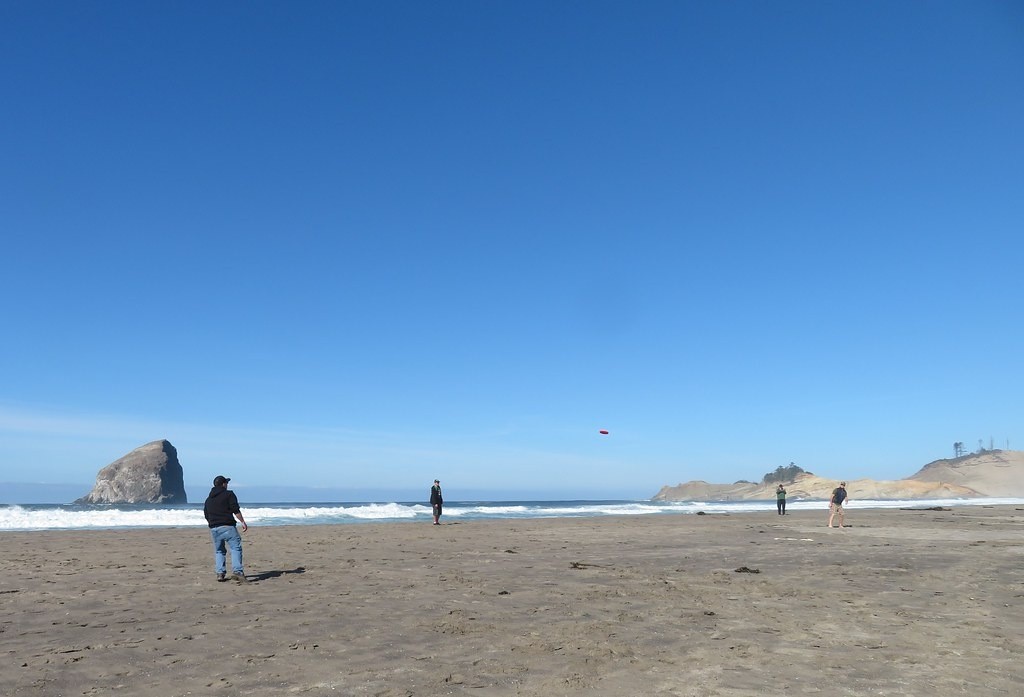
[213,476,231,486]
[840,481,846,486]
[434,479,440,483]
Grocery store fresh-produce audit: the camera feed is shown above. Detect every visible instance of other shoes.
[434,522,439,525]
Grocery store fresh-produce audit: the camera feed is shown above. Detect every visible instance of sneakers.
[217,572,225,580]
[231,571,247,582]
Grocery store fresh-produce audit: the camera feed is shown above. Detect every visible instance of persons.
[204,476,250,583]
[829,481,848,529]
[776,484,786,516]
[430,479,443,525]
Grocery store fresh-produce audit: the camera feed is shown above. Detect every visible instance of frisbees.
[600,430,609,434]
